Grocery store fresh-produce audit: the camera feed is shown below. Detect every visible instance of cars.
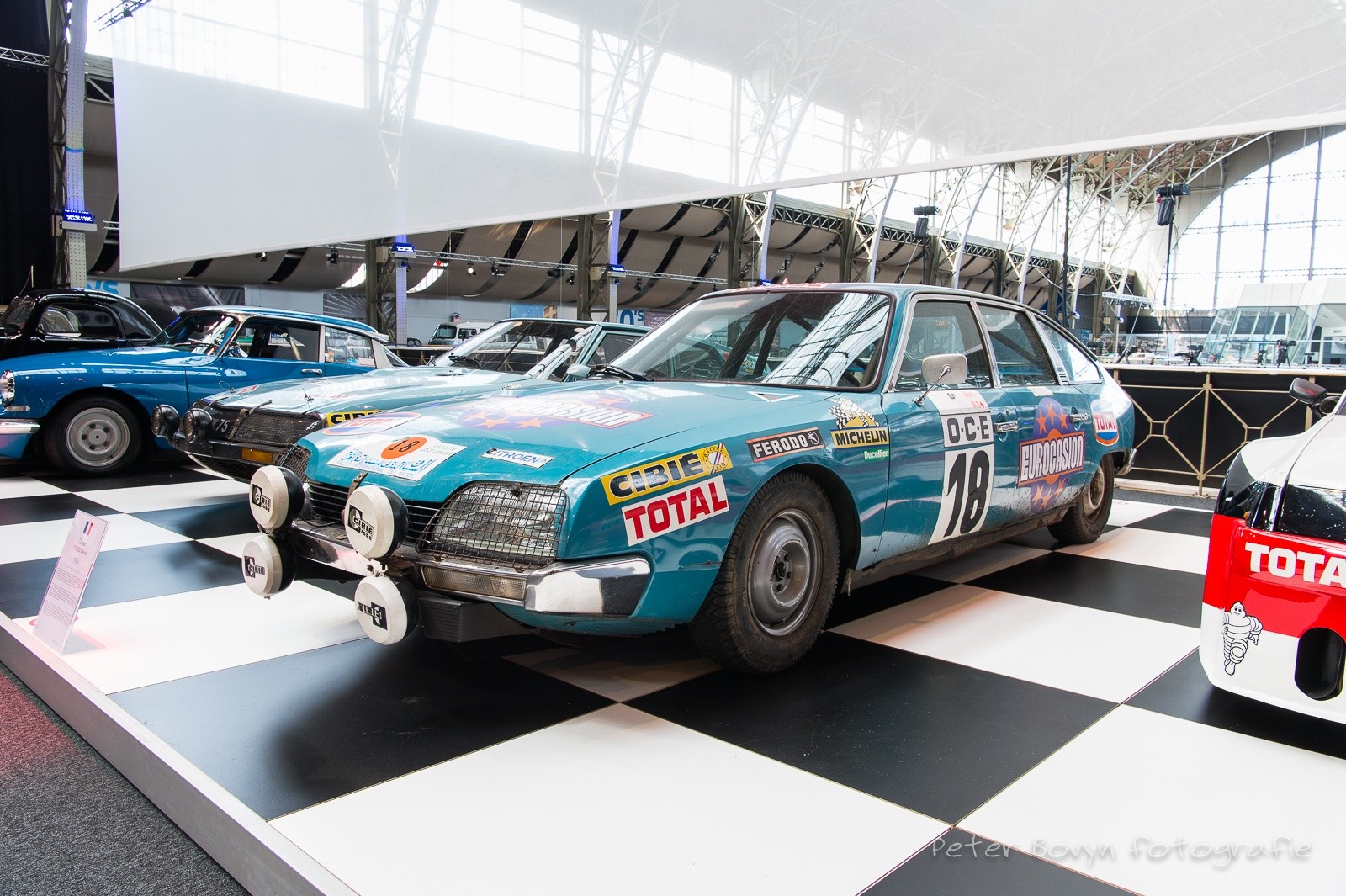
[1198,376,1346,728]
[0,288,180,362]
[253,280,1136,674]
[399,336,430,357]
[151,318,773,482]
[0,307,414,475]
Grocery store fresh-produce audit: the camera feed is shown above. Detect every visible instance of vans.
[429,321,538,352]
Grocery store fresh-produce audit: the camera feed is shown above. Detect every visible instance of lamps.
[466,262,476,277]
[565,271,575,285]
[491,264,501,276]
[634,277,642,291]
[328,251,339,264]
[547,269,564,279]
[256,253,267,262]
[712,283,718,291]
